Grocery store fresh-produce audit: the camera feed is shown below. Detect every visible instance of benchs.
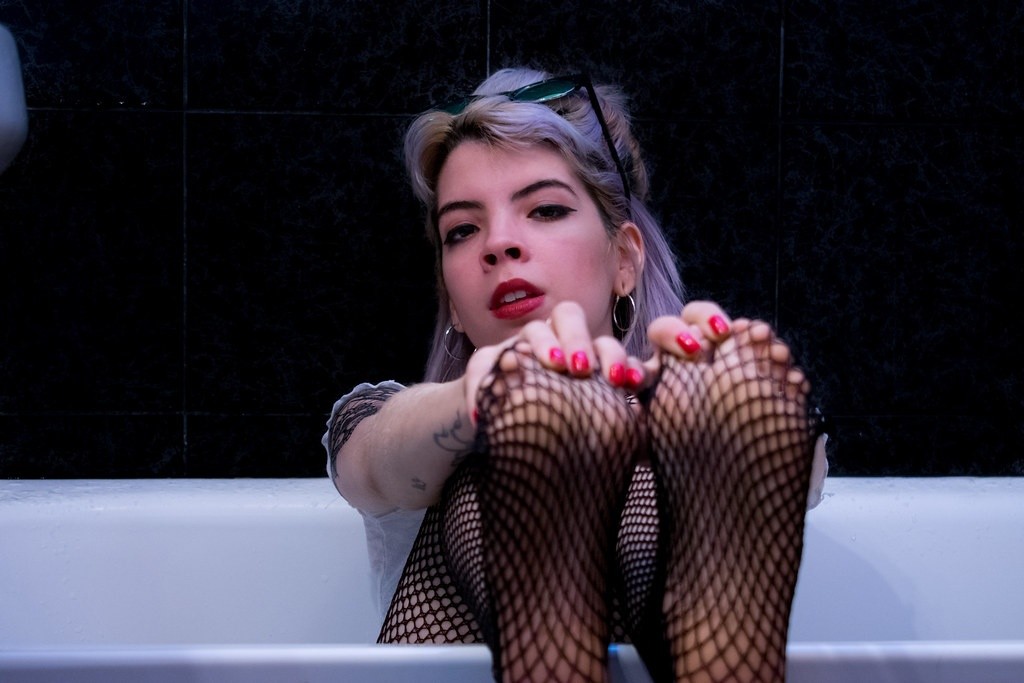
[0,476,1023,683]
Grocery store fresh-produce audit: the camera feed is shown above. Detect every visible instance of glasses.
[437,71,632,212]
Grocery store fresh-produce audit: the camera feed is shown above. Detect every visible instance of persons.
[322,65,829,683]
